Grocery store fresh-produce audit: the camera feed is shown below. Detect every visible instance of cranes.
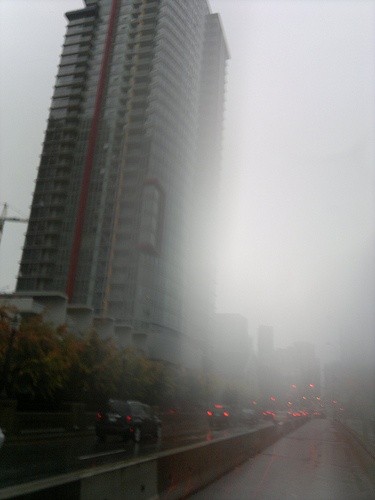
[0,203,29,241]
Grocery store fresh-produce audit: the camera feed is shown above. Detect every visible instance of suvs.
[96,398,160,442]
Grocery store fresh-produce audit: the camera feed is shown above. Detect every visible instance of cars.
[208,405,325,428]
